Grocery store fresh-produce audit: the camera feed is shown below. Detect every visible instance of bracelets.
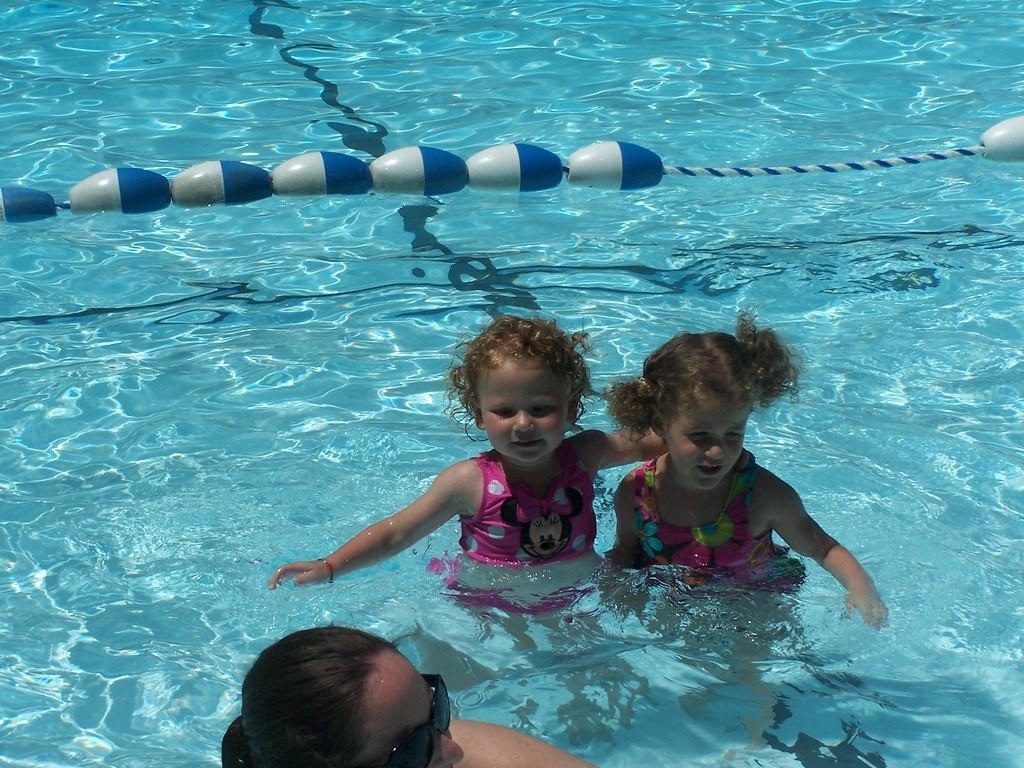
[315,558,334,584]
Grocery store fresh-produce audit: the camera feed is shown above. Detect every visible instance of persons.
[263,311,668,613]
[592,311,891,631]
[217,624,616,768]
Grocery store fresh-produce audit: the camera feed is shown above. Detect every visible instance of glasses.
[382,672,452,768]
